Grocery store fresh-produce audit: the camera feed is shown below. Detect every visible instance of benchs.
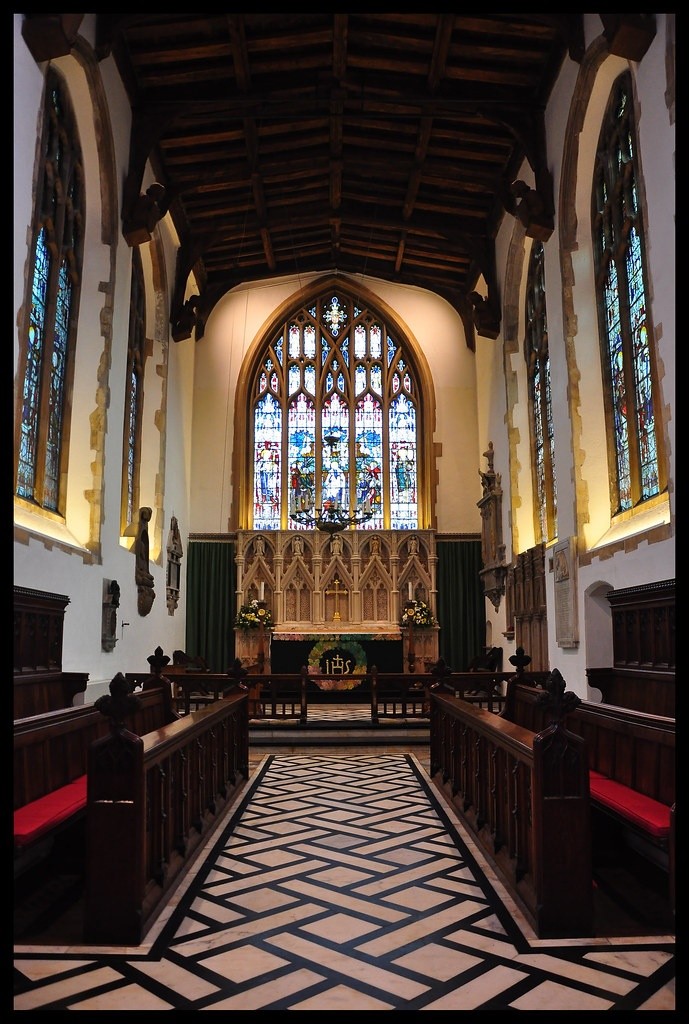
[514,683,676,917]
[14,688,164,855]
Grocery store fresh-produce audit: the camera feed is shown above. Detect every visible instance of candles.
[261,582,264,600]
[408,582,412,600]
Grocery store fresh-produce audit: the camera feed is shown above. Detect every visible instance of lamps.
[289,436,372,540]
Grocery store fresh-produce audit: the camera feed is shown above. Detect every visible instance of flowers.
[236,600,273,625]
[402,601,434,625]
